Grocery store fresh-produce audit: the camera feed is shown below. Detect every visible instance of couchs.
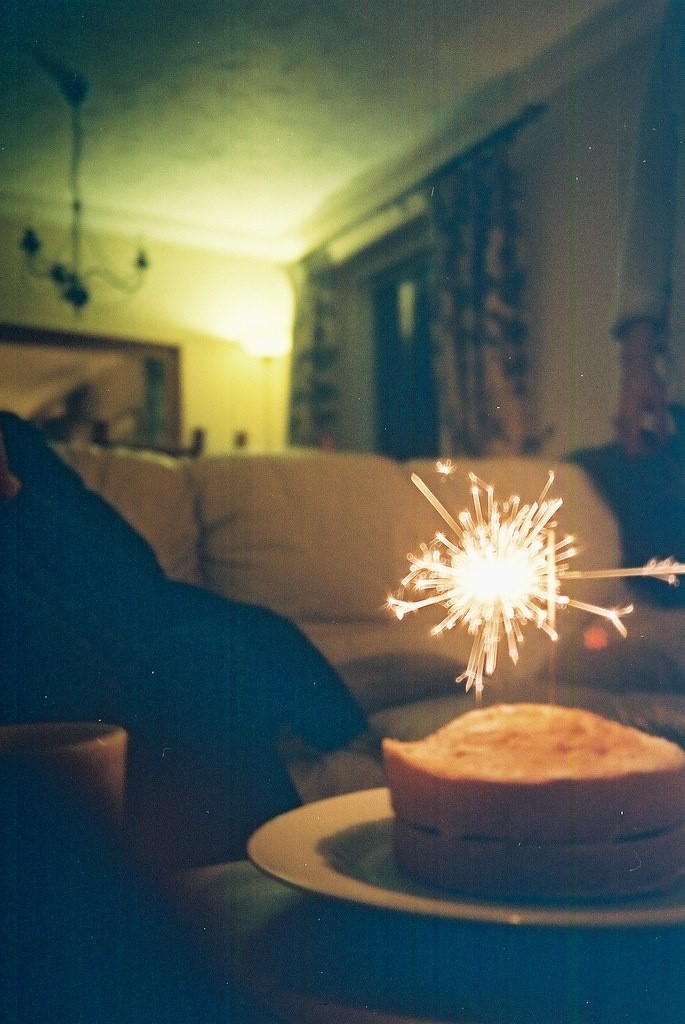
[0,445,685,798]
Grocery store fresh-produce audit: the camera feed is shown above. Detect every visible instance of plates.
[245,785,685,926]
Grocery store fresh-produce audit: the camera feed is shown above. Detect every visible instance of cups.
[0,722,127,839]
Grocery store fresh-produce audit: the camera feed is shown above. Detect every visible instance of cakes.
[382,702,685,898]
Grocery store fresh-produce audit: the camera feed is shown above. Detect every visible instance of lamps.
[19,75,152,321]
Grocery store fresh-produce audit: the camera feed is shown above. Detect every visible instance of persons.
[0,411,380,866]
[614,0,685,455]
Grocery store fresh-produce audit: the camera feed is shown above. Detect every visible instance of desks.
[0,860,685,1024]
